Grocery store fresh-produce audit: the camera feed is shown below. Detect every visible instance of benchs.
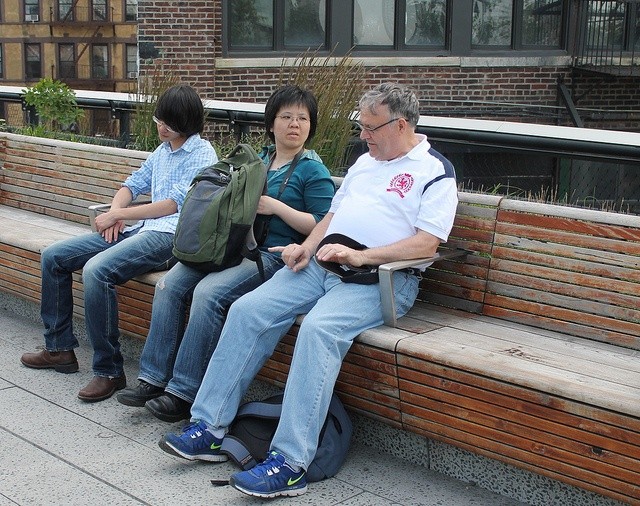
[396,198,640,491]
[1,132,501,506]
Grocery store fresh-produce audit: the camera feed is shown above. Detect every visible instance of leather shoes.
[77,373,126,401]
[117,382,166,406]
[21,350,79,373]
[145,393,192,422]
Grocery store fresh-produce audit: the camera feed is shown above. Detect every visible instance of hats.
[314,233,380,284]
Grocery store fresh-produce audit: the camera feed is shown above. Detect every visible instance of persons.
[21,85,222,402]
[115,84,338,423]
[157,81,459,499]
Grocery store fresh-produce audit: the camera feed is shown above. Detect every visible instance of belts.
[400,267,422,280]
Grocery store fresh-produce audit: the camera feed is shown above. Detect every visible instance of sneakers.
[230,452,307,498]
[158,421,228,461]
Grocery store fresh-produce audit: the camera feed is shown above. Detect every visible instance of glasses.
[354,119,407,133]
[152,117,179,134]
[274,115,311,124]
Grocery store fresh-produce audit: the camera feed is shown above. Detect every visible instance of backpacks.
[172,144,268,282]
[210,390,353,487]
